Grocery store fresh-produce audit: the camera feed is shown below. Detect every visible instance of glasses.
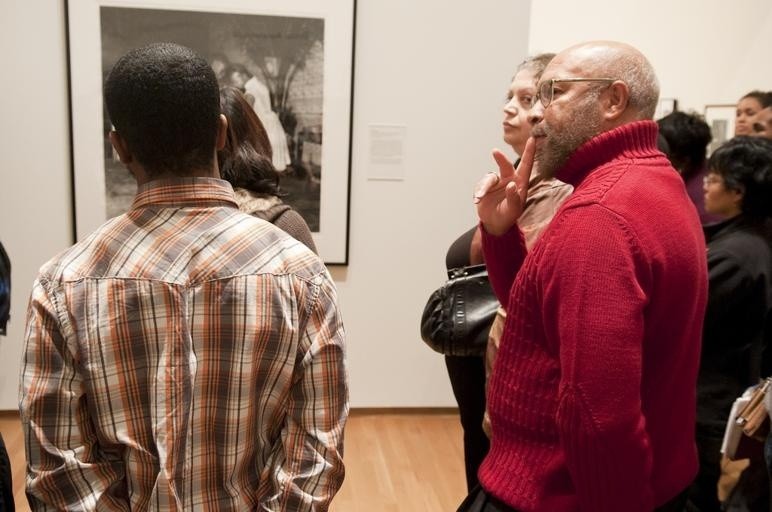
[531,77,615,108]
[702,175,726,183]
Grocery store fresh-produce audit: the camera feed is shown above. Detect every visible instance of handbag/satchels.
[420,263,504,358]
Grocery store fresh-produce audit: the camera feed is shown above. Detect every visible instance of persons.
[217,87,322,257]
[20,42,349,512]
[471,44,710,510]
[1,241,15,512]
[445,50,573,495]
[650,81,771,511]
[206,50,293,189]
